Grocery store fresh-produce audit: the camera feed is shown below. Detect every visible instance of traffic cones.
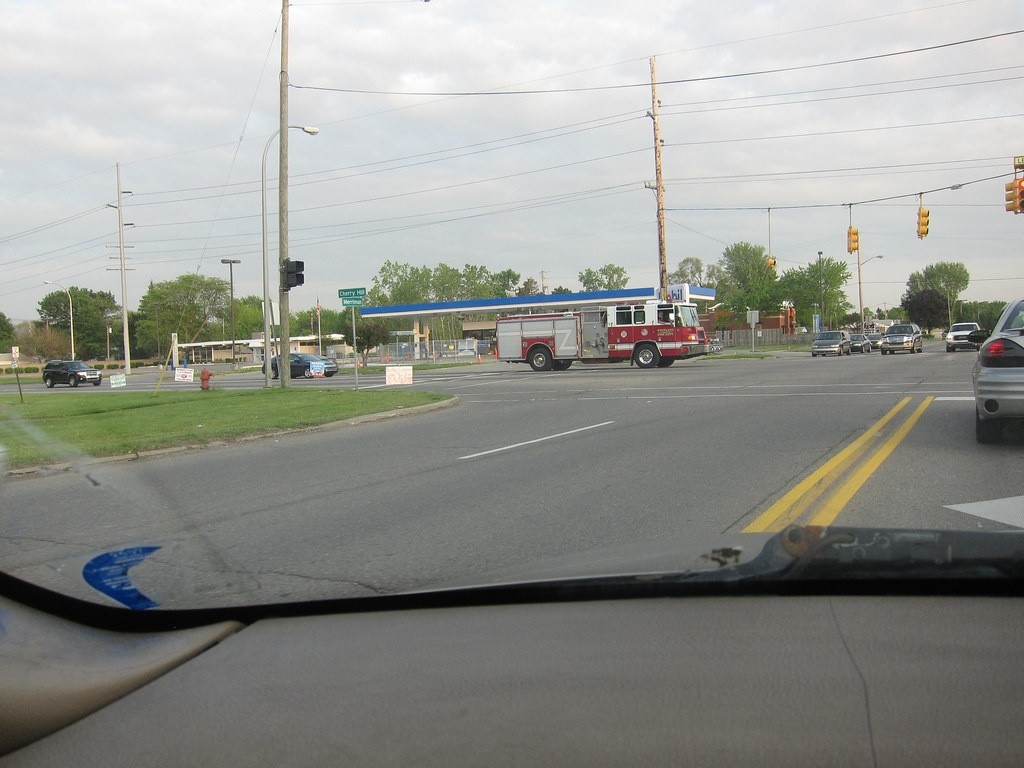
[478,354,482,362]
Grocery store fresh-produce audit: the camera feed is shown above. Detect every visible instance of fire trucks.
[494,300,725,371]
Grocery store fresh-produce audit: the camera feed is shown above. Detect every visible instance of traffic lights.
[847,227,859,254]
[768,259,776,268]
[1005,178,1024,214]
[284,259,305,288]
[917,207,930,237]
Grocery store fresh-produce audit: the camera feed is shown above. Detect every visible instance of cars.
[946,323,981,352]
[849,334,871,353]
[867,333,883,349]
[261,353,337,380]
[968,299,1024,443]
[812,330,852,357]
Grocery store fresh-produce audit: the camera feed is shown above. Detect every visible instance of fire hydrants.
[199,367,213,390]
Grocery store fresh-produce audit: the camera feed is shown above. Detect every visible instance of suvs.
[42,359,103,388]
[880,324,923,355]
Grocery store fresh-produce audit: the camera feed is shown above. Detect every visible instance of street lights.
[858,256,883,333]
[221,259,241,364]
[44,281,74,361]
[262,126,319,388]
